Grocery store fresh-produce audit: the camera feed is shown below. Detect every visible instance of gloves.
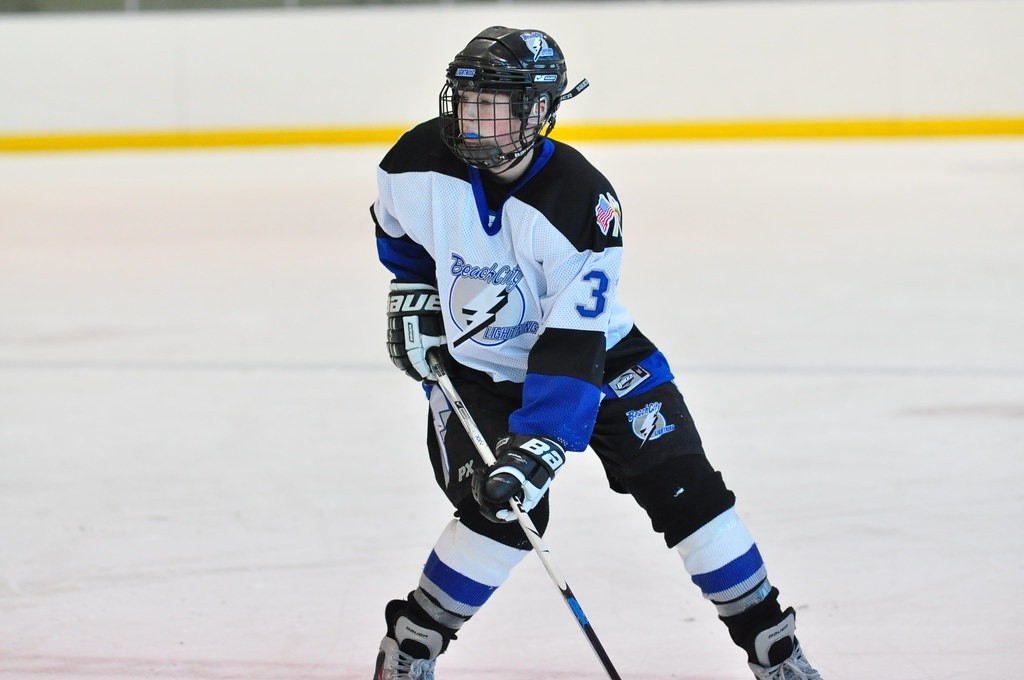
[465,440,565,523]
[386,290,450,385]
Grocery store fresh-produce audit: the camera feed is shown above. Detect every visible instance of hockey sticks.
[427,358,624,680]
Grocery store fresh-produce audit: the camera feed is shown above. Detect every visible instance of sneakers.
[374,600,456,680]
[745,605,825,679]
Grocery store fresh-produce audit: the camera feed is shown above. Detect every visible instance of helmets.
[438,26,570,167]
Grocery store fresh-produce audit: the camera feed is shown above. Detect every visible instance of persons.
[370,26,821,680]
